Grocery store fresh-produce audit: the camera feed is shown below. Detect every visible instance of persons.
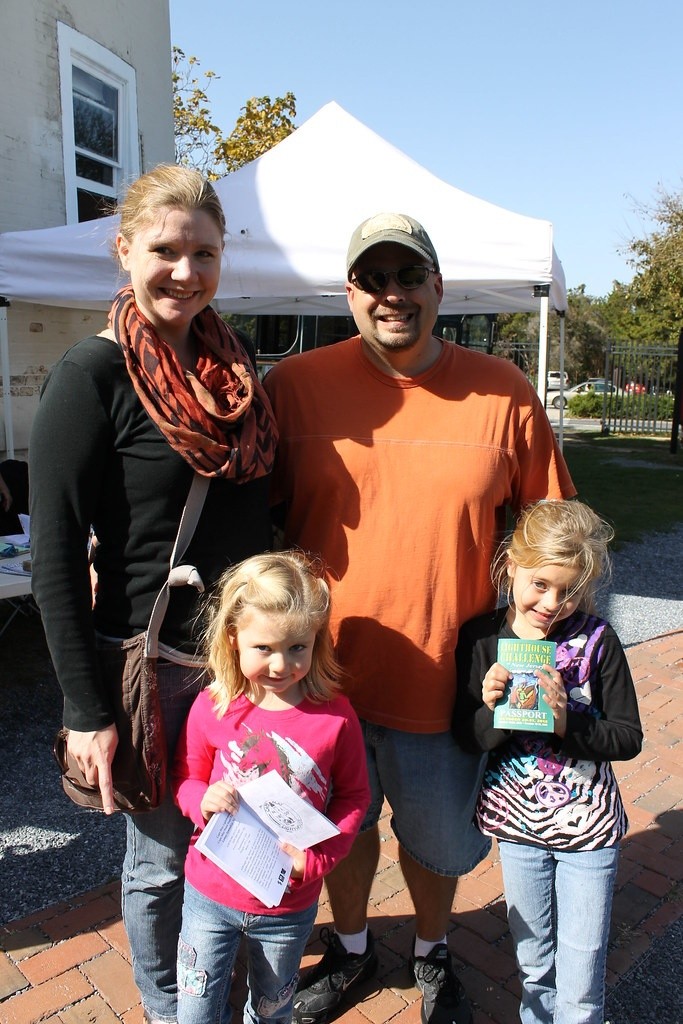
[262,214,577,1024]
[448,501,643,1024]
[29,166,283,1023]
[170,553,371,1024]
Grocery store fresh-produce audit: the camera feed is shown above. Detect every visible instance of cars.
[586,378,610,391]
[625,383,644,394]
[546,382,628,409]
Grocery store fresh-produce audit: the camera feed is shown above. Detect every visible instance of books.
[494,637,557,733]
[193,769,341,909]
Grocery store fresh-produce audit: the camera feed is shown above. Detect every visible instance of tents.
[0,100,566,451]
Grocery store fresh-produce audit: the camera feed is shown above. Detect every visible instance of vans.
[547,370,571,391]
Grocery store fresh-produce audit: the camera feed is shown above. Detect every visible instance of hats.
[347,213,439,280]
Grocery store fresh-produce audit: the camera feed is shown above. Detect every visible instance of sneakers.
[290,928,376,1024]
[409,933,473,1024]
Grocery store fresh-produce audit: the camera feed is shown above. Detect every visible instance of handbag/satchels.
[61,633,166,813]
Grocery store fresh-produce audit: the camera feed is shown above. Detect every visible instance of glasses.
[348,266,436,293]
